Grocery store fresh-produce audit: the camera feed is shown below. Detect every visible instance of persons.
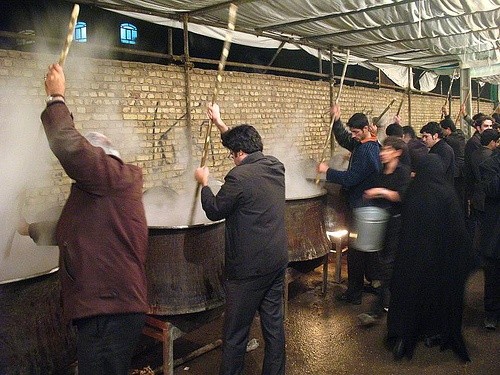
[18,61,152,375]
[379,111,500,362]
[194,103,288,375]
[318,103,385,303]
[378,136,409,183]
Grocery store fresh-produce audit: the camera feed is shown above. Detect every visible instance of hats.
[86,132,125,166]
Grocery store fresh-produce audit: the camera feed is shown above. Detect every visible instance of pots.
[147,218,225,229]
[285,190,327,200]
[0,266,59,285]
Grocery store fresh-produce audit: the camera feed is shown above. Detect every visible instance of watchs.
[44,95,65,103]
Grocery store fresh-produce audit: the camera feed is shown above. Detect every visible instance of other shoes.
[359,312,378,324]
[337,293,363,305]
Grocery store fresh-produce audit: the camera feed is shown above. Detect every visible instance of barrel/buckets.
[347,205,390,252]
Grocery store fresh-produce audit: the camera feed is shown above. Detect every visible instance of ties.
[227,150,236,160]
